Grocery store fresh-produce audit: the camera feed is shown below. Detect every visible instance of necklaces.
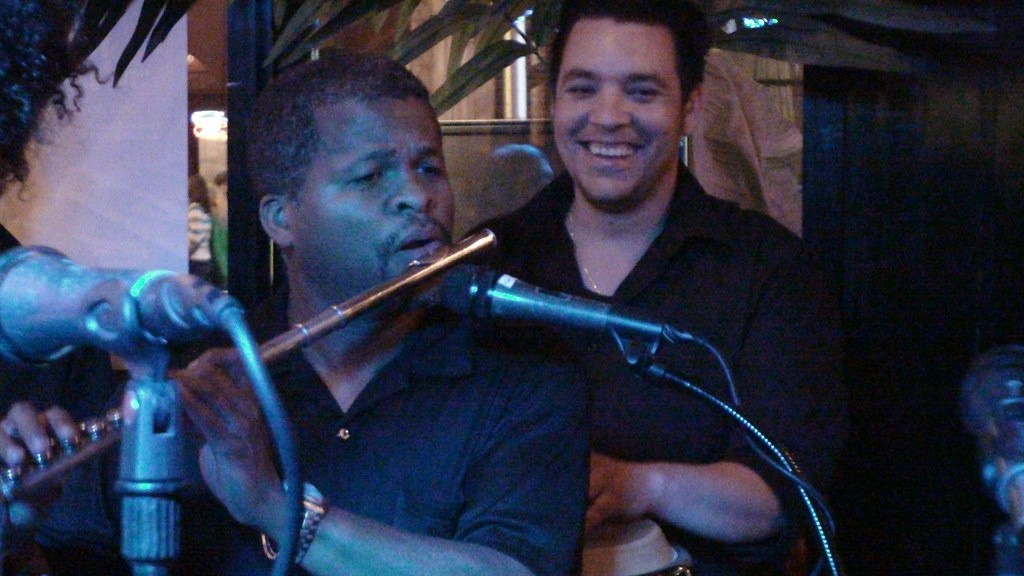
[566,214,601,294]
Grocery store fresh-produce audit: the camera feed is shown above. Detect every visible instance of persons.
[963,344,1024,550]
[0,51,595,576]
[456,1,848,576]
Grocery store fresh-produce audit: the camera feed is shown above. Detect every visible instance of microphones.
[959,344,1024,525]
[442,265,705,349]
[0,245,247,367]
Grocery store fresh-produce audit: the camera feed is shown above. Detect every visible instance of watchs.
[261,476,331,565]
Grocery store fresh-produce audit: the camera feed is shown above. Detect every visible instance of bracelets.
[993,463,1023,512]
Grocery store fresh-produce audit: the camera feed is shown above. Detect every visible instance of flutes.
[0,223,500,505]
